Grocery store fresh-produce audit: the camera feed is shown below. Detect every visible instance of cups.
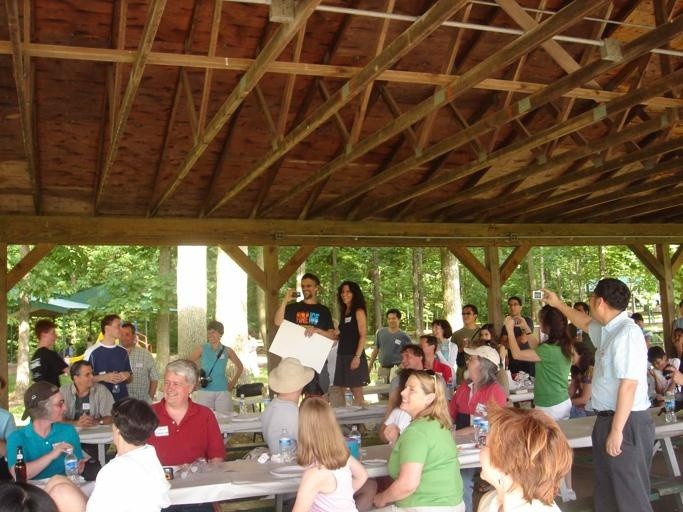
[346,439,360,459]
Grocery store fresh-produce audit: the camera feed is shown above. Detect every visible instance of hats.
[269,357,314,394]
[21,381,59,421]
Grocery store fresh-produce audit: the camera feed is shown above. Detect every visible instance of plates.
[362,459,388,465]
[270,466,304,477]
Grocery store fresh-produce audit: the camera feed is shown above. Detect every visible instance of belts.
[594,410,615,418]
[381,365,393,368]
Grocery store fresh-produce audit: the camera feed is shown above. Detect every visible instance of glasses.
[506,303,521,308]
[477,435,491,450]
[587,293,600,298]
[462,312,475,315]
[463,346,501,370]
[423,366,437,394]
[46,397,65,410]
[72,360,86,372]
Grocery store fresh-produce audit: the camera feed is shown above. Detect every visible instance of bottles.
[14,447,27,483]
[180,457,207,482]
[447,377,455,400]
[279,427,291,463]
[390,365,399,382]
[576,328,583,343]
[64,448,80,487]
[473,406,488,448]
[344,387,353,409]
[239,394,246,416]
[349,425,362,447]
[664,390,676,423]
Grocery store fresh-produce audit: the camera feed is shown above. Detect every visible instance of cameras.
[663,363,677,380]
[163,467,173,480]
[512,319,517,325]
[532,291,545,300]
[292,292,301,297]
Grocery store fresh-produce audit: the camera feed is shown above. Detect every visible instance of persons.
[374,369,466,511]
[0,379,16,479]
[369,296,682,443]
[273,272,336,395]
[537,277,655,511]
[7,381,86,479]
[475,408,573,512]
[82,314,159,404]
[326,278,374,435]
[291,397,368,511]
[261,357,377,509]
[58,360,116,425]
[145,359,225,510]
[0,481,57,510]
[192,320,243,413]
[28,320,73,389]
[43,398,171,510]
